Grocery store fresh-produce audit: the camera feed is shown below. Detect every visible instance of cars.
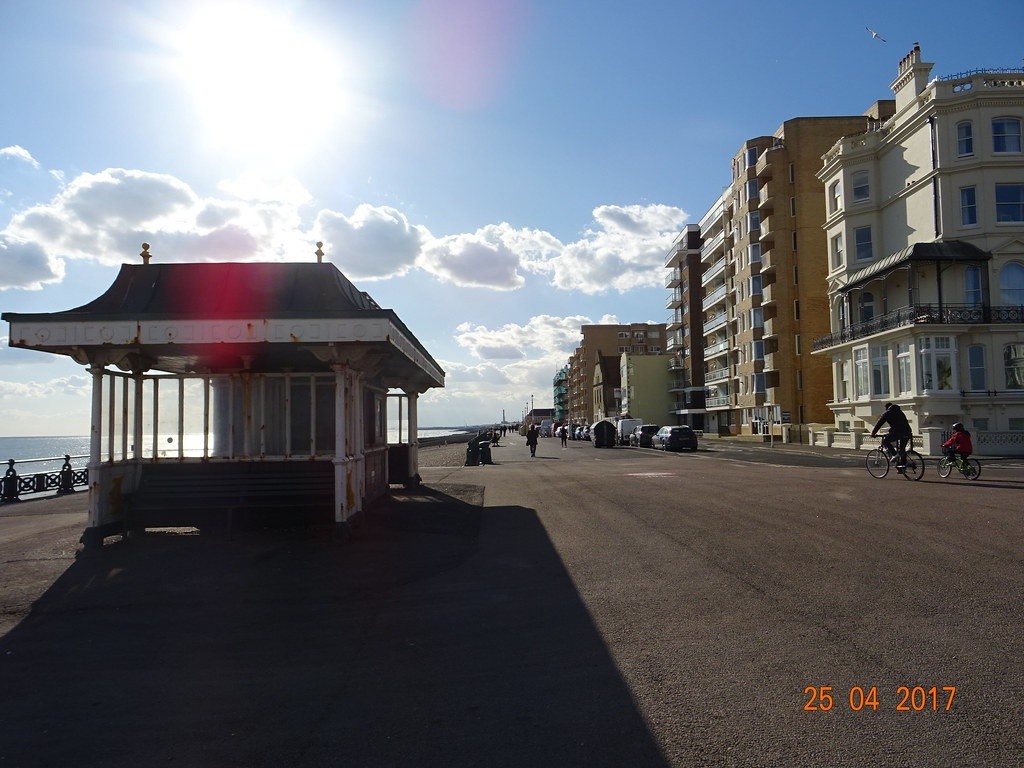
[538,417,642,446]
[650,425,698,453]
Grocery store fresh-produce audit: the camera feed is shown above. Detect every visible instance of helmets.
[953,422,964,430]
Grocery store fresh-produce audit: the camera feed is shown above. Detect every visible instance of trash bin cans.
[486,432,492,440]
[479,441,492,464]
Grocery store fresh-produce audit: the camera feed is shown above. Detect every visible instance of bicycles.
[936,446,982,481]
[865,433,925,481]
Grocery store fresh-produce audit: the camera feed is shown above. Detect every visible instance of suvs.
[628,424,660,448]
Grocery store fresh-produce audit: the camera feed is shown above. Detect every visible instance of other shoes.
[531,454,535,457]
[890,453,899,462]
[961,465,970,470]
[945,461,956,467]
[897,467,906,474]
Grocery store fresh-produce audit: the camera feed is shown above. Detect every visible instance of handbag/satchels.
[526,441,529,446]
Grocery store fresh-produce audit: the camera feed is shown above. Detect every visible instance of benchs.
[124,460,335,535]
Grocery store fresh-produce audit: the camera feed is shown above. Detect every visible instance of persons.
[487,424,518,437]
[493,433,499,447]
[871,402,912,474]
[560,425,567,448]
[445,439,447,447]
[527,425,538,458]
[942,423,972,470]
[439,443,440,448]
